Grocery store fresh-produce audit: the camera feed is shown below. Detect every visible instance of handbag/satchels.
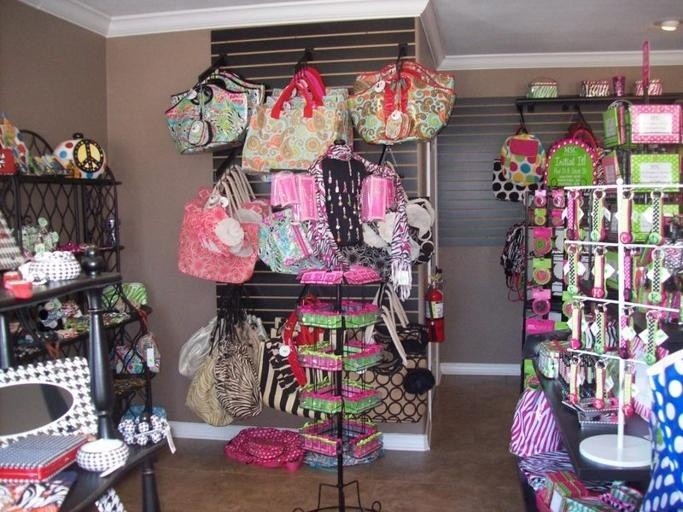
[224,427,307,468]
[100,282,148,324]
[500,223,526,276]
[368,323,428,376]
[107,332,160,380]
[178,310,333,427]
[509,390,576,492]
[493,133,547,203]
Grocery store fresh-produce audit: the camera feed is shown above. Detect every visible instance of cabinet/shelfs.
[0,125,159,453]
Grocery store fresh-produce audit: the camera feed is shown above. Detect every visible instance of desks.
[0,424,175,512]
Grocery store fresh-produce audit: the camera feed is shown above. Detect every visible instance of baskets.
[29,250,81,281]
